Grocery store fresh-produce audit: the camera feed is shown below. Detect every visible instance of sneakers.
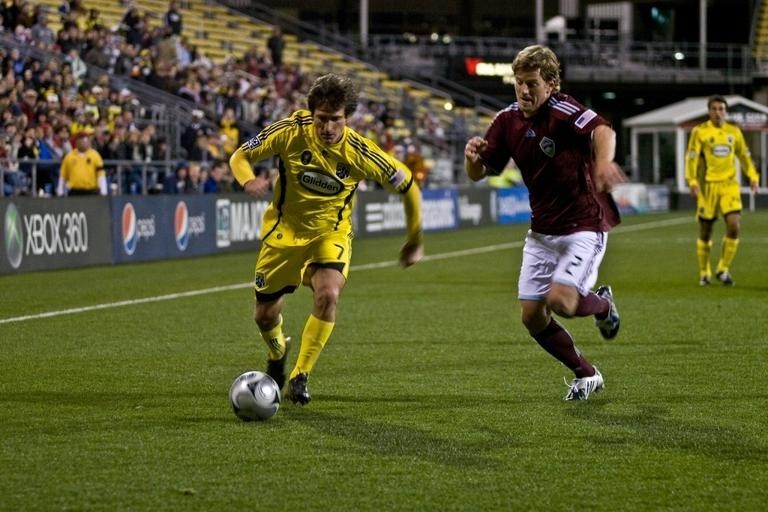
[563,366,606,403]
[263,339,288,391]
[595,285,621,341]
[699,271,712,286]
[716,266,734,286]
[289,374,314,404]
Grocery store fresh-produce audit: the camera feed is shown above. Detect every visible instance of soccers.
[228,371,283,423]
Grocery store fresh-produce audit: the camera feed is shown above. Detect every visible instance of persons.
[55,134,107,196]
[465,45,628,400]
[685,95,759,286]
[0,0,453,194]
[229,73,424,405]
